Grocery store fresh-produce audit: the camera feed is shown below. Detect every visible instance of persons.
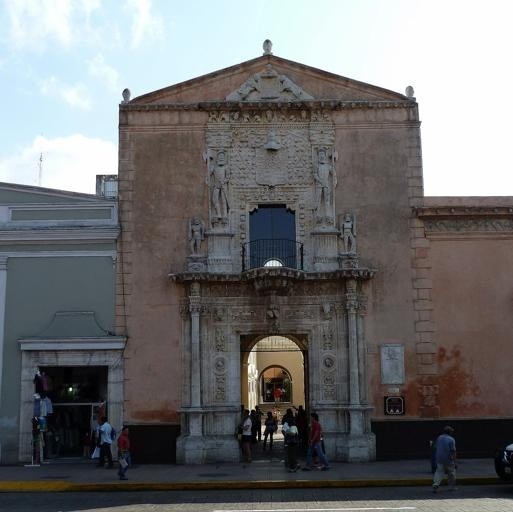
[206,149,230,217]
[189,217,205,254]
[239,385,331,472]
[431,426,457,493]
[340,214,356,253]
[117,427,130,480]
[430,429,444,474]
[97,416,113,469]
[313,146,338,225]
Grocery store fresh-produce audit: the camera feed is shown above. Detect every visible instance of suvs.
[494,438,513,482]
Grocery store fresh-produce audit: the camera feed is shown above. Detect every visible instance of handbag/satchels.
[110,427,116,440]
